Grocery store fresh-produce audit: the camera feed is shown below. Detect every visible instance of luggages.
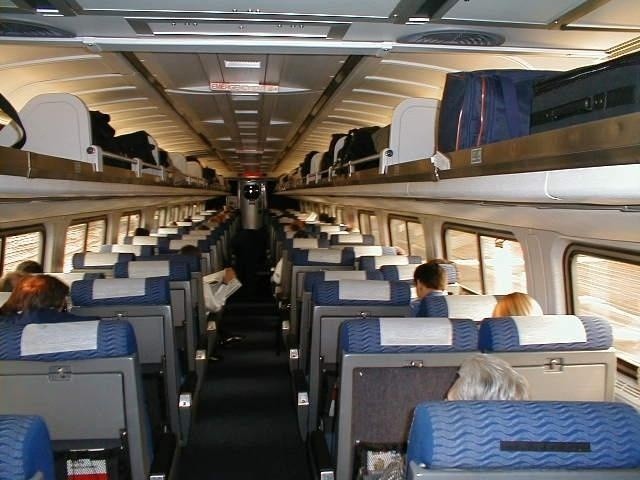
[276,150,324,189]
[0,94,230,191]
[437,51,640,154]
[325,123,390,174]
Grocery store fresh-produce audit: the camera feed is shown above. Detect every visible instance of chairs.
[0,209,240,479]
[263,206,640,480]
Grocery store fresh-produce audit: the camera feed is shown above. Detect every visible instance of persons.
[411,262,448,305]
[492,292,543,317]
[135,203,236,311]
[444,354,528,401]
[270,212,355,284]
[0,260,69,313]
